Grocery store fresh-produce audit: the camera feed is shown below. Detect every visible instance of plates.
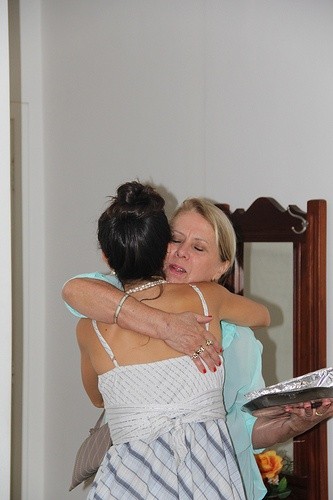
[240,386,333,414]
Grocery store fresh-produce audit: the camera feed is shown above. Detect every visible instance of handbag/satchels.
[68,423,112,492]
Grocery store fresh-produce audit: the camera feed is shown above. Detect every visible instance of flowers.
[254,450,291,500]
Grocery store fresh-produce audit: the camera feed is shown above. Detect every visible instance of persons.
[76,181,270,500]
[60,198,333,500]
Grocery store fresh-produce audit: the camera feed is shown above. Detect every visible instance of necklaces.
[125,280,170,294]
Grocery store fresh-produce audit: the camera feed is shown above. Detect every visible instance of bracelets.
[114,294,128,328]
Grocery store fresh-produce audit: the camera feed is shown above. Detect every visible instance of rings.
[190,353,200,360]
[197,345,206,355]
[314,409,324,418]
[205,340,215,347]
